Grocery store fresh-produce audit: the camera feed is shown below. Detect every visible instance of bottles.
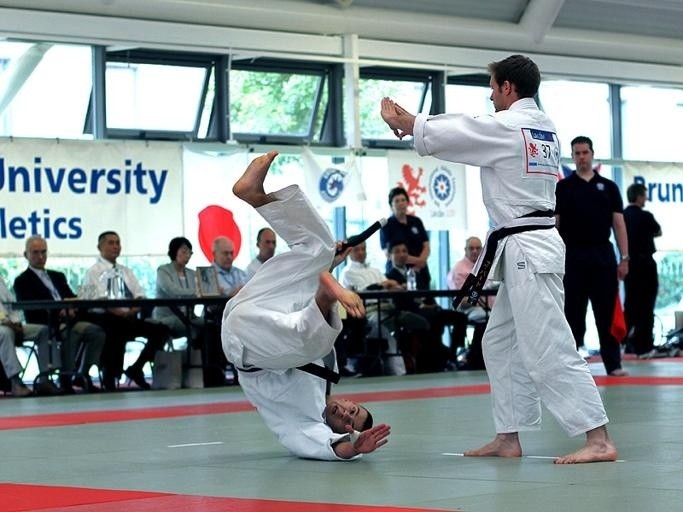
[408,266,415,291]
[101,265,126,300]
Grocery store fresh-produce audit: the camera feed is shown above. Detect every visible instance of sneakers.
[11,375,31,396]
[101,367,118,391]
[38,379,61,395]
[60,374,77,394]
[124,365,151,389]
[76,374,101,394]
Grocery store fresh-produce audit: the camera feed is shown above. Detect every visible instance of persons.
[623,183,662,361]
[380,55,617,464]
[1,186,494,395]
[555,137,631,376]
[220,151,390,461]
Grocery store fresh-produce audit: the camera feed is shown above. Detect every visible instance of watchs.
[622,255,631,261]
